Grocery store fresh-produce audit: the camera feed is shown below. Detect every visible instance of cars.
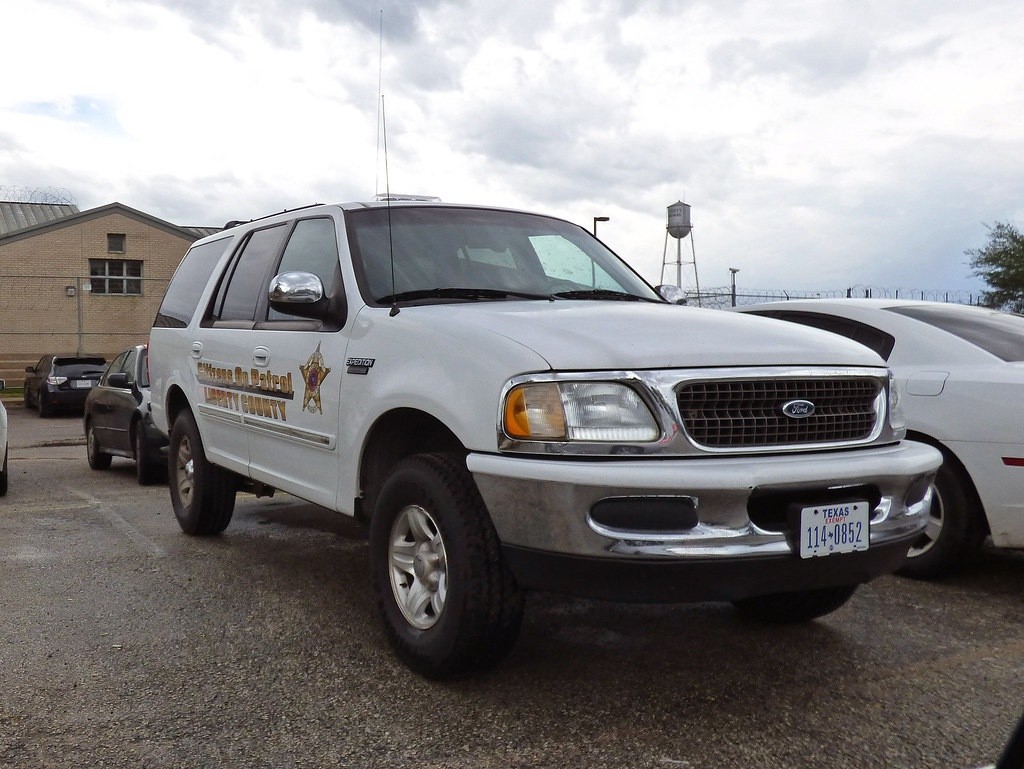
[721,297,1024,578]
[0,377,9,499]
[81,345,170,486]
[23,353,109,418]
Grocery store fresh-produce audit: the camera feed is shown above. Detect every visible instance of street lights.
[591,216,611,288]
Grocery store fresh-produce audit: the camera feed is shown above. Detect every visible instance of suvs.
[147,192,944,684]
